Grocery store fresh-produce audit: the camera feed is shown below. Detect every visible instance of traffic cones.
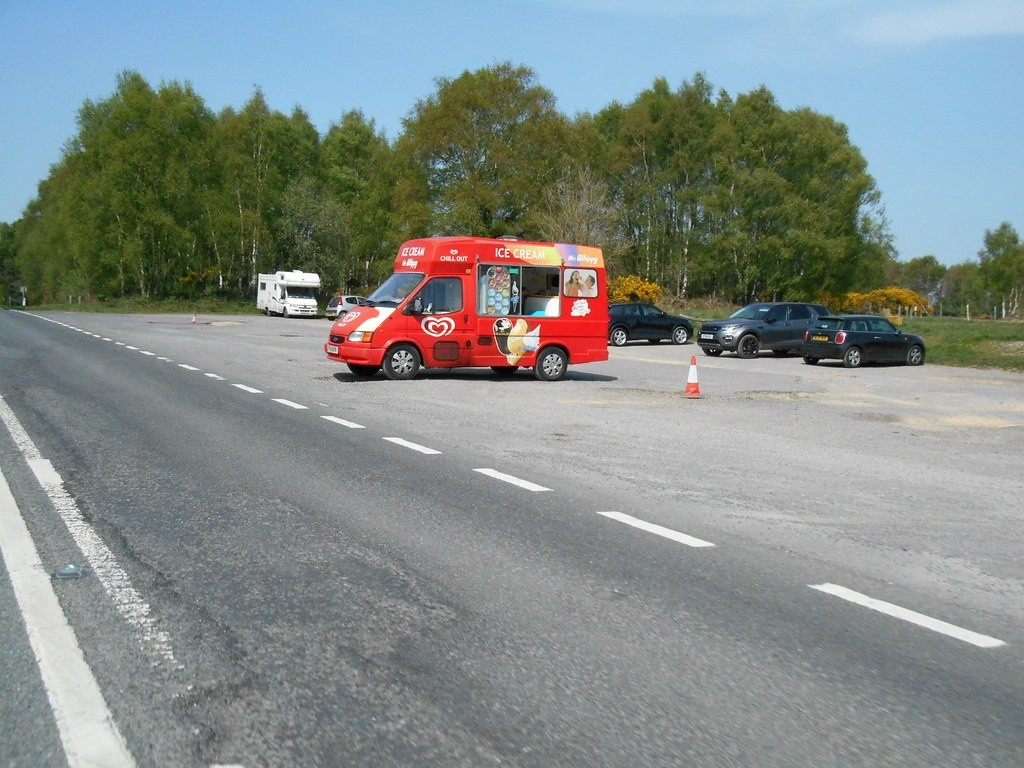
[192,312,197,323]
[684,356,702,398]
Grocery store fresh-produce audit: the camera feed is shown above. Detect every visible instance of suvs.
[607,302,695,347]
[696,302,830,359]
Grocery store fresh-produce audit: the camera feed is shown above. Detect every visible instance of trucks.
[256,270,321,317]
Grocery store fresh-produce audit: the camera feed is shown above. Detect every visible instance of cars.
[324,296,367,321]
[801,315,926,367]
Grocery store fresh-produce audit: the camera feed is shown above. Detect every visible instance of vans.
[322,235,610,382]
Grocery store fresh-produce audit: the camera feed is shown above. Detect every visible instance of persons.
[395,282,409,299]
[565,271,596,297]
